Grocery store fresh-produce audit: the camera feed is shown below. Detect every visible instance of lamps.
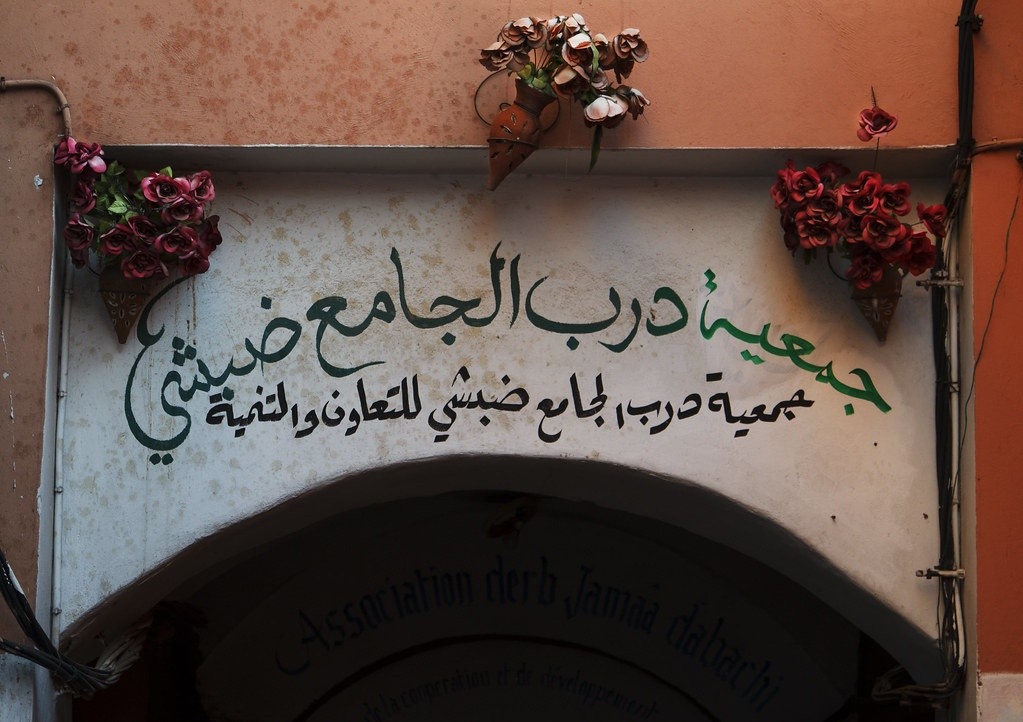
[485,497,537,539]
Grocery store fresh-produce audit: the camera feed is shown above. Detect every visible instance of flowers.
[479,12,653,175]
[770,86,940,286]
[52,135,223,284]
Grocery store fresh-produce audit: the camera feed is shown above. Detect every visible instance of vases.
[851,270,902,344]
[485,77,558,193]
[98,268,151,344]
